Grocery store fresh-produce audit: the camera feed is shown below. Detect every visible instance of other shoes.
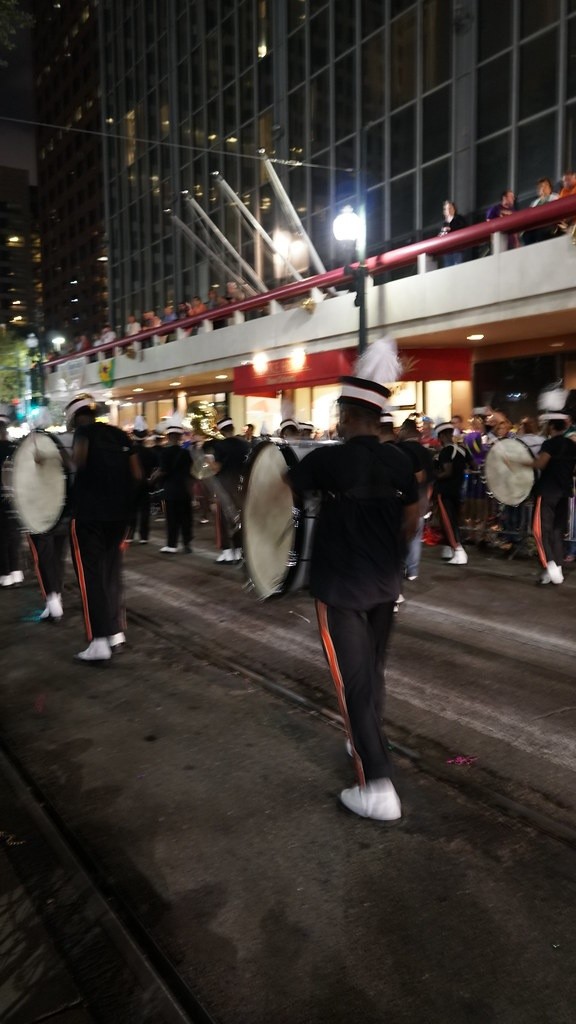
[439,543,454,559]
[9,570,25,588]
[70,640,112,666]
[0,575,15,589]
[125,536,245,567]
[447,545,469,565]
[153,517,166,522]
[536,561,564,585]
[39,596,65,624]
[343,736,360,761]
[199,518,210,524]
[335,783,405,828]
[406,575,419,580]
[106,631,127,648]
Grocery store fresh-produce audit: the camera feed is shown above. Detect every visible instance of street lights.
[331,202,368,356]
[27,332,65,405]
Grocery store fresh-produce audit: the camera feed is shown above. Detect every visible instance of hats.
[336,333,405,414]
[164,419,185,435]
[536,388,570,421]
[432,417,456,435]
[298,421,315,435]
[129,416,149,441]
[151,425,165,439]
[215,417,234,431]
[279,418,299,436]
[61,393,98,433]
[0,414,12,425]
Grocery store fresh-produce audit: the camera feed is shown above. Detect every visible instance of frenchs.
[181,400,226,479]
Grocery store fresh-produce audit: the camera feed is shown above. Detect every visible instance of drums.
[480,433,547,509]
[2,430,76,539]
[236,436,343,605]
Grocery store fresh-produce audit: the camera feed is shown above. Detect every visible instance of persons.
[382,389,576,584]
[286,339,420,821]
[0,415,70,619]
[49,297,207,372]
[125,416,337,563]
[521,170,576,245]
[437,201,466,267]
[65,397,136,659]
[487,191,519,250]
[209,281,245,330]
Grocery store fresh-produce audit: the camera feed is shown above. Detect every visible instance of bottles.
[462,466,469,488]
